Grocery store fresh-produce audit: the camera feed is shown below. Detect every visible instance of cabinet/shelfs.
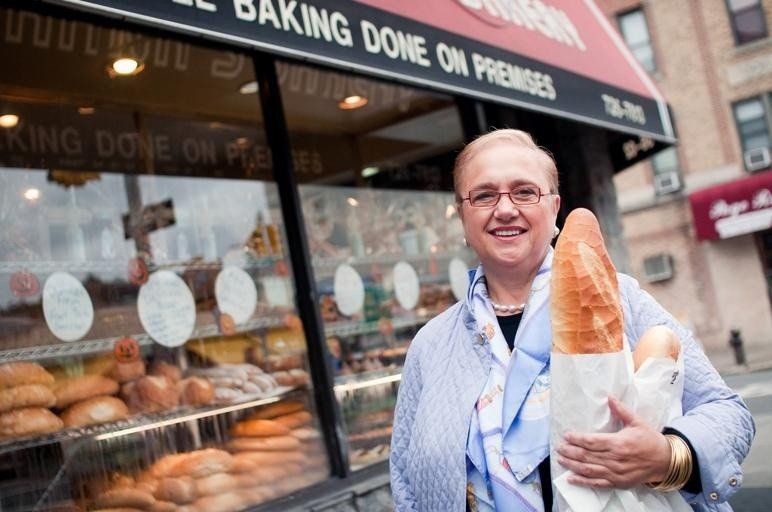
[0,249,462,512]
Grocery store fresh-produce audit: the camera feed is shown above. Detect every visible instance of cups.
[462,186,552,207]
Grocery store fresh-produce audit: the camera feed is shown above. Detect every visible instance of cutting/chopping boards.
[729,329,746,365]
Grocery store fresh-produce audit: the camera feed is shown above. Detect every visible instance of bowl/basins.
[489,299,527,313]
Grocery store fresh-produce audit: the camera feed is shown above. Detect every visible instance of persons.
[389,126,758,512]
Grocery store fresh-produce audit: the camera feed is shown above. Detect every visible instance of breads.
[0,357,326,512]
[633,326,681,373]
[550,208,623,354]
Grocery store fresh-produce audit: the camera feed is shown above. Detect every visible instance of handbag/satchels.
[641,433,693,494]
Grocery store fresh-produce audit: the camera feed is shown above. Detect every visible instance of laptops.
[550,208,623,354]
[633,326,681,373]
